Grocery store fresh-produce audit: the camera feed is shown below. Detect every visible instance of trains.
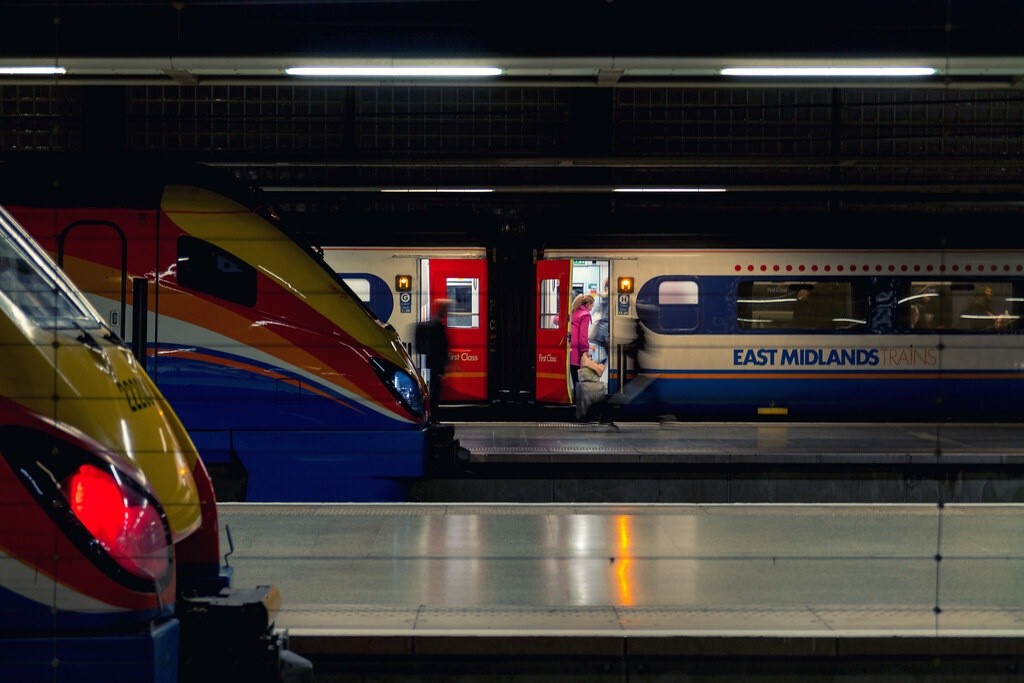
[0,160,440,682]
[302,246,1024,423]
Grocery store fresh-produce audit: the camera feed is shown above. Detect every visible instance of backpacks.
[415,322,429,356]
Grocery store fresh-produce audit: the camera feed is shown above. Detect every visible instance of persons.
[966,285,1012,333]
[909,291,941,329]
[425,297,453,427]
[788,284,833,328]
[552,278,609,405]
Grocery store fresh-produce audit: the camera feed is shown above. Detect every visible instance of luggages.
[575,381,607,422]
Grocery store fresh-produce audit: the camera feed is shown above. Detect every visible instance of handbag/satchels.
[579,352,607,377]
[586,310,604,339]
